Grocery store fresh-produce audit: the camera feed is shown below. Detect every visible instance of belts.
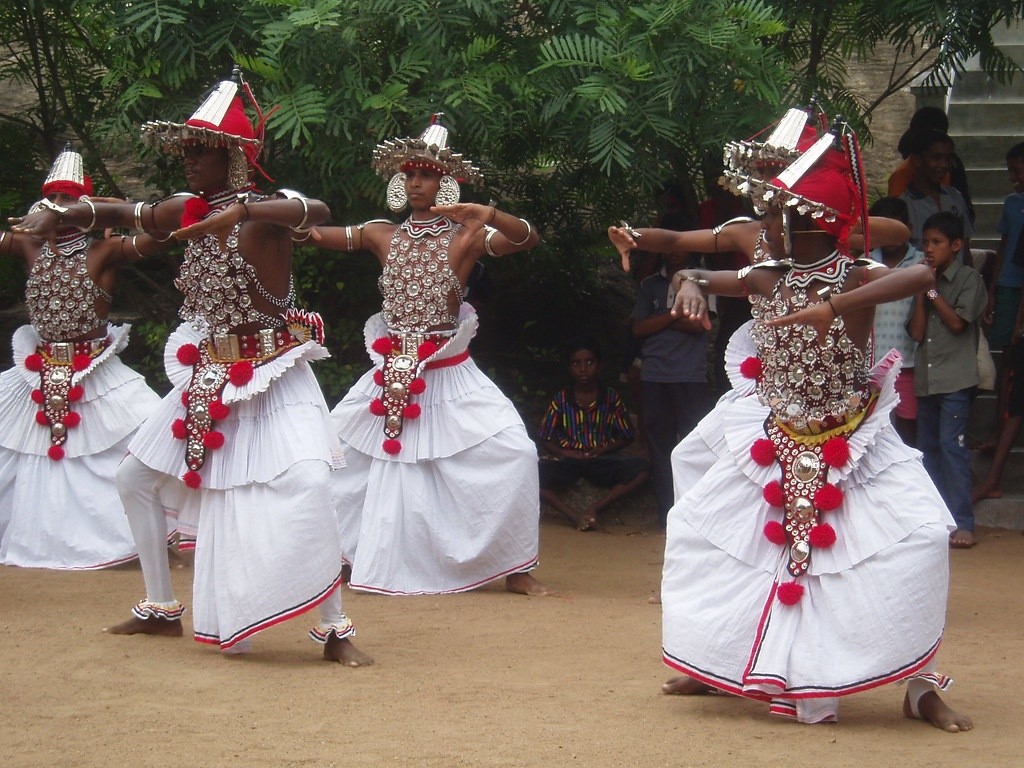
[388,331,449,357]
[39,338,112,365]
[207,328,300,360]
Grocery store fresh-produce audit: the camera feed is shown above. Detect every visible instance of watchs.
[927,288,939,301]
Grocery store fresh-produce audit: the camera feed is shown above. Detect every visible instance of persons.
[631,208,716,528]
[861,193,989,550]
[970,222,1024,503]
[663,118,975,735]
[541,334,651,531]
[308,123,564,600]
[975,142,1024,453]
[9,80,373,668]
[888,105,979,259]
[609,110,911,604]
[0,151,196,570]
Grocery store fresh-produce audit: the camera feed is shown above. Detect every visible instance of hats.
[371,111,486,213]
[723,115,871,260]
[716,92,829,207]
[140,65,282,190]
[42,140,95,200]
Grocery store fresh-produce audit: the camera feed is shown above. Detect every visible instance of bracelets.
[288,195,308,230]
[508,219,531,246]
[39,196,69,225]
[822,293,838,317]
[135,199,146,233]
[238,200,249,221]
[486,198,496,225]
[77,201,95,232]
[0,232,6,244]
[677,271,709,289]
[620,220,642,239]
